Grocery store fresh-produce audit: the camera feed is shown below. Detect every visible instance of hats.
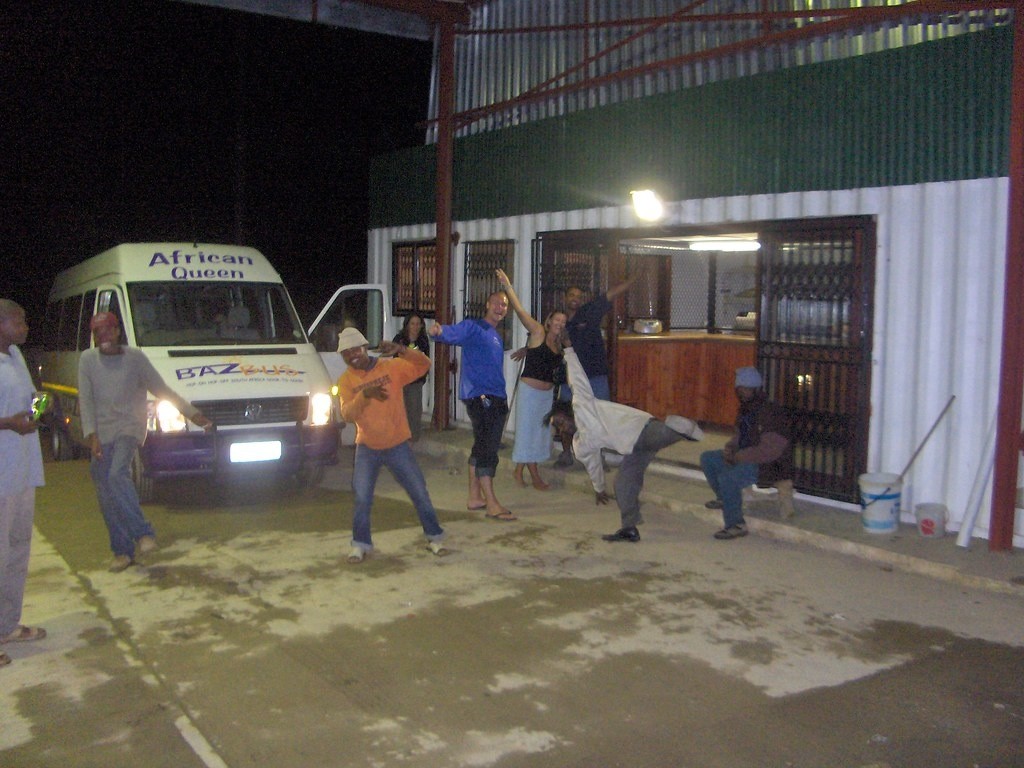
[337,327,368,353]
[733,366,761,388]
[89,311,120,329]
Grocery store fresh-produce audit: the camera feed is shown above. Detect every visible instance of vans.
[37,242,393,505]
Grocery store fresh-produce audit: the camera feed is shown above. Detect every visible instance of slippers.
[485,510,517,522]
[467,503,487,511]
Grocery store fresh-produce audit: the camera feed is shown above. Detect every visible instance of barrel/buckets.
[914,503,950,538]
[858,472,902,534]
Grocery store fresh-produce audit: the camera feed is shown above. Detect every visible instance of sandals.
[0,625,47,645]
[0,653,11,667]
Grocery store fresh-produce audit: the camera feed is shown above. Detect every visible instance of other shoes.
[602,457,610,473]
[704,500,723,510]
[426,540,450,556]
[136,535,160,554]
[713,523,747,540]
[107,553,134,573]
[347,546,367,564]
[554,452,573,469]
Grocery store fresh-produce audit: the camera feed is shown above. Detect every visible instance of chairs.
[134,301,185,345]
[219,306,260,339]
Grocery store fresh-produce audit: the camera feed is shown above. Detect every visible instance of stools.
[771,479,795,519]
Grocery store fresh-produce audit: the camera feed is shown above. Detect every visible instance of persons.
[390,313,430,442]
[0,298,53,665]
[329,326,449,564]
[427,290,519,522]
[699,366,797,540]
[323,317,357,351]
[544,336,706,543]
[495,266,568,490]
[511,274,637,474]
[78,310,214,573]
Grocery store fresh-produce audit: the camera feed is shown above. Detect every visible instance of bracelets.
[399,338,402,354]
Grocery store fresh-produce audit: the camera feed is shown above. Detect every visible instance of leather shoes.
[665,415,703,443]
[601,527,640,543]
